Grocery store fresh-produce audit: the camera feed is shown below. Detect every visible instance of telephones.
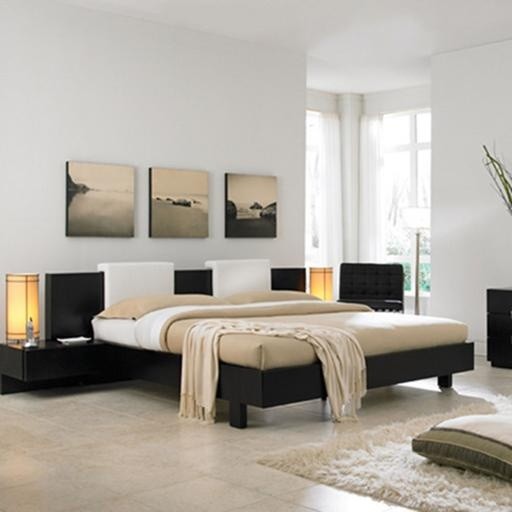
[24,320,38,348]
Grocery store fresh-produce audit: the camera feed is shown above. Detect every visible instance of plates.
[57,336,92,345]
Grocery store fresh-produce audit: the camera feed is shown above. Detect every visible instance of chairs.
[335,262,404,314]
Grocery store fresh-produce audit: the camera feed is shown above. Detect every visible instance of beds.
[46,256,477,430]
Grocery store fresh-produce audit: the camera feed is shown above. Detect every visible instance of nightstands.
[2,334,112,398]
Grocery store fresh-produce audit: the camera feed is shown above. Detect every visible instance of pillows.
[221,289,322,304]
[410,409,511,485]
[91,292,229,322]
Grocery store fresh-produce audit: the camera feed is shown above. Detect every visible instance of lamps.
[308,265,334,301]
[4,270,41,342]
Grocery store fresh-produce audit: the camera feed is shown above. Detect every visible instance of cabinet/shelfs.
[485,287,511,371]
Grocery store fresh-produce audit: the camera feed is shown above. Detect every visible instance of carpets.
[254,392,511,512]
